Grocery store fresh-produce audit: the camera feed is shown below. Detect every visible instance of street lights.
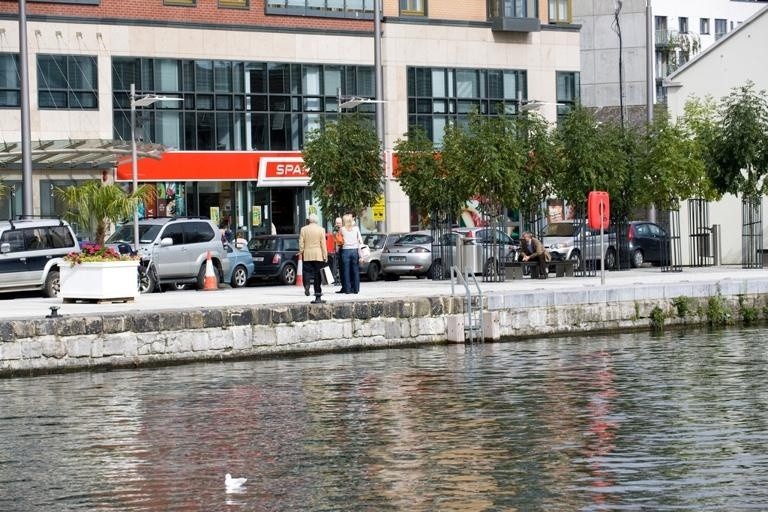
[335,86,384,232]
[127,83,186,250]
[521,100,565,117]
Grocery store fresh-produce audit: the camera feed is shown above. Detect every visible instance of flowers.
[65,240,140,268]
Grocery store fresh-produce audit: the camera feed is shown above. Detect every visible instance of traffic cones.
[201,249,219,290]
[294,253,303,285]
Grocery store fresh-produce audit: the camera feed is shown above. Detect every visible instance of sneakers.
[542,271,549,280]
[305,286,360,297]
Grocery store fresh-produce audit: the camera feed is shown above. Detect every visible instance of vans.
[539,219,620,272]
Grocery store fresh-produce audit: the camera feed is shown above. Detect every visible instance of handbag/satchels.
[319,263,335,286]
[357,244,371,264]
[335,231,345,248]
[543,250,552,262]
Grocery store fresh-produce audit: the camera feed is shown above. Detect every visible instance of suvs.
[97,214,229,294]
[0,213,82,300]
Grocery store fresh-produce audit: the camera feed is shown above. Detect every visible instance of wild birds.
[223,473,249,489]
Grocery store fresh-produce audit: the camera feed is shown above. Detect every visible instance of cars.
[247,234,338,285]
[171,235,255,291]
[612,220,670,268]
[359,227,520,282]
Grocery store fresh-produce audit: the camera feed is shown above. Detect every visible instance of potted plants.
[51,180,158,298]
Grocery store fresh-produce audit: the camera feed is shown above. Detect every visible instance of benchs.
[501,258,576,280]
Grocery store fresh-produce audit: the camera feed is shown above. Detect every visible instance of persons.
[336,213,362,294]
[215,216,278,251]
[297,212,329,296]
[517,230,551,280]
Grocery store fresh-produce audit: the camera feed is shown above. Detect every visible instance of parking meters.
[325,233,337,284]
